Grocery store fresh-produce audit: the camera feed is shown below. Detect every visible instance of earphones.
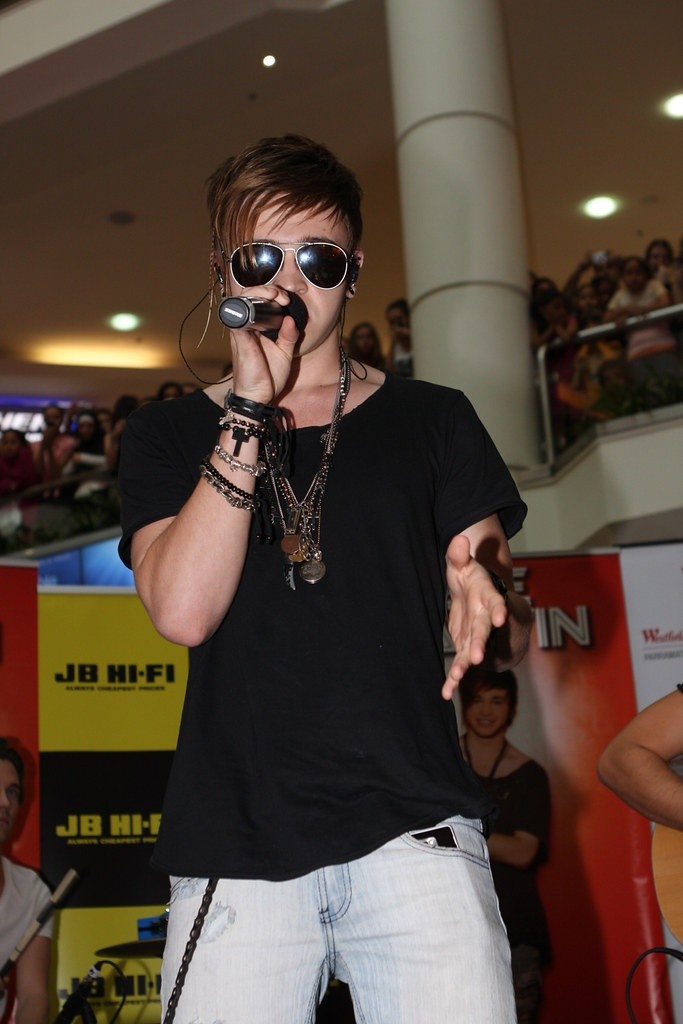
[346,260,359,285]
[213,264,225,285]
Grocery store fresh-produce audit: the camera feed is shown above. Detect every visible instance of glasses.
[222,240,352,292]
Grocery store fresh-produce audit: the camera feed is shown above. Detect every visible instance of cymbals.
[93,937,166,960]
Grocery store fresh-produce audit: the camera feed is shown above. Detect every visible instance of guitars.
[649,822,683,948]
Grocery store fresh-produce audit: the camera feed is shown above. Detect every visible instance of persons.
[339,238,683,449]
[456,663,550,1024]
[1,736,56,1024]
[119,133,530,1024]
[596,683,683,831]
[0,381,195,545]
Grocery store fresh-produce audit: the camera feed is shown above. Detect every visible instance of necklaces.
[463,733,508,778]
[255,354,352,591]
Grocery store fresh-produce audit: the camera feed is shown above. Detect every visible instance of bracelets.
[198,459,256,512]
[218,413,269,457]
[224,388,275,423]
[214,446,267,477]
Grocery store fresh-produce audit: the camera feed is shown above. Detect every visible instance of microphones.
[218,290,308,334]
[53,963,104,1024]
